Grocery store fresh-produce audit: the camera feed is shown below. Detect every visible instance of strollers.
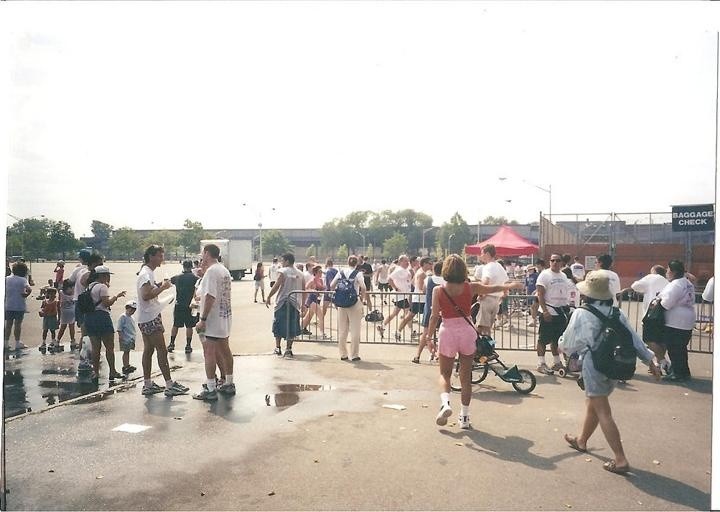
[556,335,589,377]
[442,293,538,395]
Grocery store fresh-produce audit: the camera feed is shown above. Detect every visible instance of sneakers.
[141,381,166,396]
[191,383,219,401]
[435,401,452,426]
[4,338,198,381]
[536,362,555,376]
[273,326,422,365]
[551,360,567,372]
[164,381,190,396]
[219,381,237,396]
[458,414,471,428]
[647,364,690,382]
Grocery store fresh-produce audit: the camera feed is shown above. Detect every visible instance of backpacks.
[76,281,103,316]
[331,268,361,308]
[575,303,638,381]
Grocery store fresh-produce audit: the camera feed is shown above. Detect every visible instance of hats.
[574,269,617,301]
[92,266,115,275]
[124,299,137,309]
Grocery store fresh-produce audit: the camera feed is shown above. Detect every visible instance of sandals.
[564,432,587,452]
[601,457,631,474]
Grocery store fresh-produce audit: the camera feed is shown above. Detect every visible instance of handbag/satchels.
[553,305,571,320]
[641,298,667,327]
[475,334,497,357]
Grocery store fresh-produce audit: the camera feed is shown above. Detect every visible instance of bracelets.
[201,317,207,321]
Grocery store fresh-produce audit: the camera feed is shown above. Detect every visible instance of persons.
[655,260,698,383]
[329,254,368,363]
[424,252,525,429]
[4,239,229,399]
[411,261,446,366]
[556,269,662,475]
[191,244,237,402]
[615,265,671,374]
[264,251,308,358]
[584,253,623,309]
[701,276,714,304]
[533,252,574,375]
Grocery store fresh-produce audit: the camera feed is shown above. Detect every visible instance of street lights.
[355,229,365,255]
[240,201,276,263]
[476,200,512,243]
[499,175,552,225]
[447,232,456,254]
[9,214,45,255]
[422,226,435,257]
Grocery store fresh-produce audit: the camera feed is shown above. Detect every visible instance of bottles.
[195,322,208,344]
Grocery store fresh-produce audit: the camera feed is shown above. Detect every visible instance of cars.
[36,258,47,263]
[8,255,25,263]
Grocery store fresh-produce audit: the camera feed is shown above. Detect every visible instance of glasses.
[549,259,562,263]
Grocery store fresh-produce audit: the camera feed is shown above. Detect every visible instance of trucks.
[198,239,253,281]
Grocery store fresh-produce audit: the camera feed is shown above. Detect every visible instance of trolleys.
[694,314,713,336]
[486,272,531,328]
